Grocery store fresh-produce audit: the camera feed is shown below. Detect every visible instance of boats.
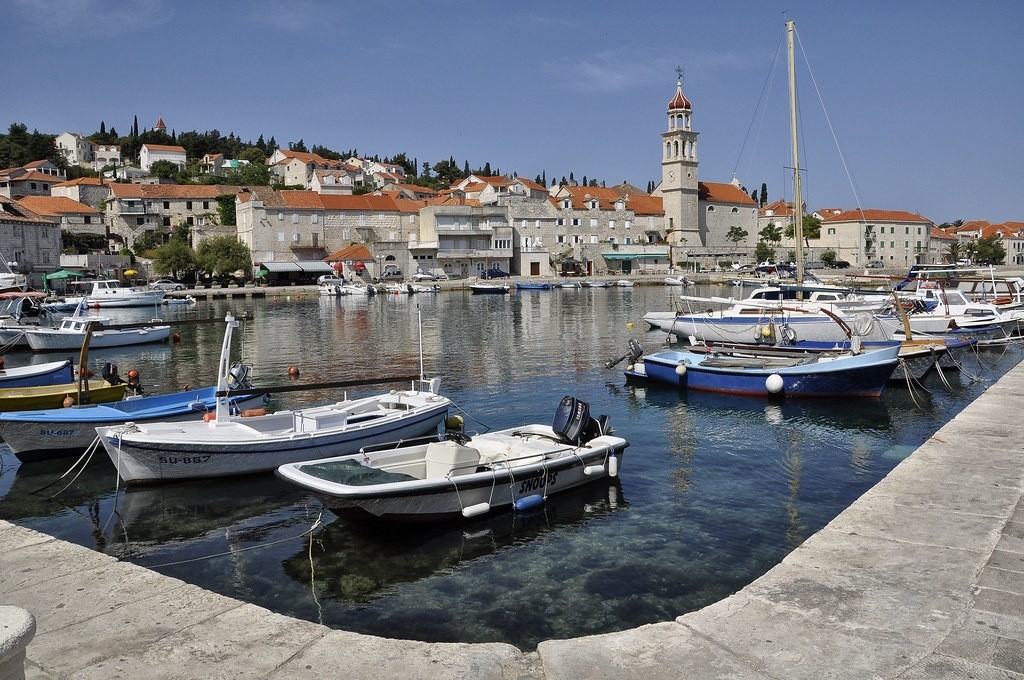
[317,276,772,296]
[91,376,452,486]
[274,394,631,533]
[1,268,275,464]
[626,271,1024,402]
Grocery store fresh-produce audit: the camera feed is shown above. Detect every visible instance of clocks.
[667,170,676,182]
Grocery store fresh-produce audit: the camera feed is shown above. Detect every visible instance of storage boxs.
[425,440,480,479]
[293,408,347,432]
